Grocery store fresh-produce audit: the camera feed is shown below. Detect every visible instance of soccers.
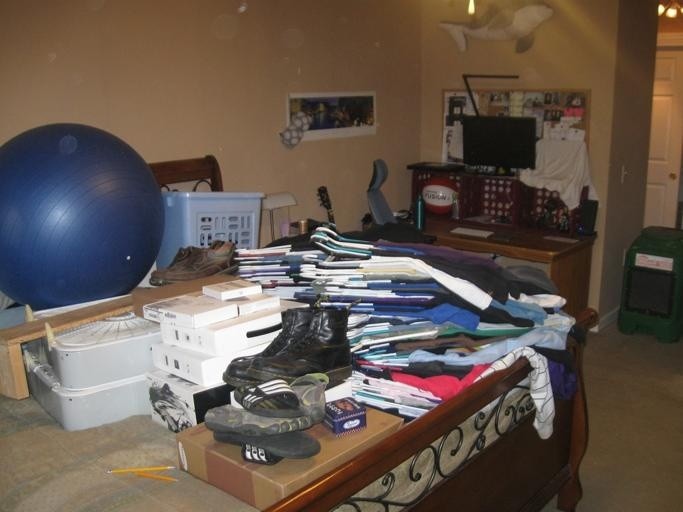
[422,177,458,215]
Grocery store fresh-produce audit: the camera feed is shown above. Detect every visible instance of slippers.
[213,427,322,467]
[234,380,303,419]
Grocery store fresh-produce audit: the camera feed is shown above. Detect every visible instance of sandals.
[149,241,238,285]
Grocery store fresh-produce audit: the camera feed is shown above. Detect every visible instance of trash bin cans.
[617,226,680,342]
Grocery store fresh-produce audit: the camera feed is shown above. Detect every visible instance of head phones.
[392,209,410,220]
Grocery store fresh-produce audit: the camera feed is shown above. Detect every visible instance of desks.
[394,216,596,318]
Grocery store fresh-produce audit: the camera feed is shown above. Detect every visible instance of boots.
[247,306,354,386]
[222,307,311,387]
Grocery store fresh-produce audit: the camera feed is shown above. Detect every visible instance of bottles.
[416,194,425,231]
[451,191,461,220]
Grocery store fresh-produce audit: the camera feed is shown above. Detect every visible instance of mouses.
[491,215,510,223]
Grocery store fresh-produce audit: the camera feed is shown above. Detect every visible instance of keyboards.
[450,226,494,239]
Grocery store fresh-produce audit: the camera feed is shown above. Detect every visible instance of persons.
[544,109,560,120]
[543,91,551,104]
[563,92,584,117]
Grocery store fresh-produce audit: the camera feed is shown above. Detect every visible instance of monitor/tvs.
[463,115,536,176]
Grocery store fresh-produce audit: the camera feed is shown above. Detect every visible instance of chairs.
[365,158,393,225]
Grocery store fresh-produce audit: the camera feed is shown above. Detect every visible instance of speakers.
[579,200,598,236]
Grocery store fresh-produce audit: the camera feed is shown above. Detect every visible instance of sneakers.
[205,373,329,439]
[149,382,191,434]
